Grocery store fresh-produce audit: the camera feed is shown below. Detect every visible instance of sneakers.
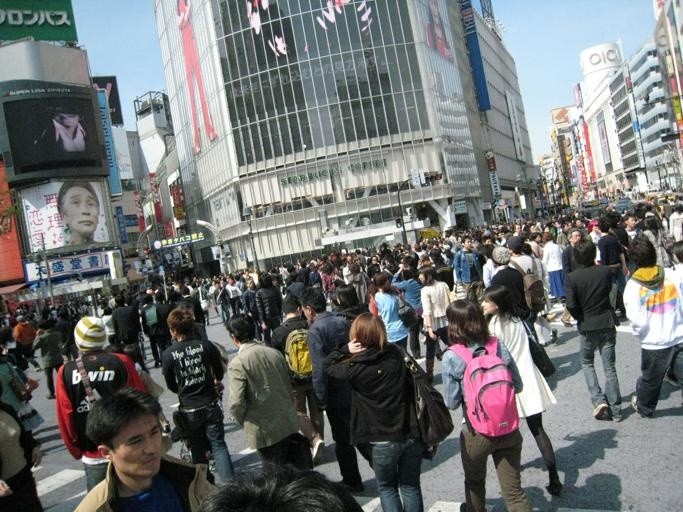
[630,394,652,418]
[155,358,160,368]
[34,367,41,373]
[312,438,326,462]
[593,403,606,417]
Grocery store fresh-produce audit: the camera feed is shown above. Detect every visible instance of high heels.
[546,469,558,492]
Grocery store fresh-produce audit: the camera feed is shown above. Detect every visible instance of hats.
[492,246,510,264]
[73,314,107,350]
[506,235,524,252]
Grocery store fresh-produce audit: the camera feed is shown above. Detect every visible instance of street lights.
[31,252,48,300]
[242,203,262,274]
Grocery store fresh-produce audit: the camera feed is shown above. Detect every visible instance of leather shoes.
[47,393,57,401]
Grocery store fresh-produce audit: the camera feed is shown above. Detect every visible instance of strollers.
[170,381,225,473]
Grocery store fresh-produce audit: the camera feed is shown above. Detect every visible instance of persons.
[391,256,429,359]
[598,218,626,321]
[440,220,500,252]
[453,236,486,303]
[323,313,454,512]
[506,236,546,343]
[561,228,588,327]
[32,320,63,399]
[624,188,683,268]
[271,294,325,466]
[0,288,117,346]
[564,240,623,423]
[163,310,233,481]
[623,234,682,419]
[116,266,281,371]
[57,181,100,246]
[369,271,418,352]
[365,239,453,280]
[199,461,363,512]
[56,317,150,494]
[225,312,314,468]
[0,356,45,439]
[111,296,149,373]
[500,202,623,244]
[542,233,566,307]
[298,288,372,492]
[271,243,371,313]
[72,387,215,512]
[483,247,555,377]
[480,285,564,495]
[0,397,44,512]
[441,299,533,512]
[330,286,370,319]
[417,265,452,383]
[13,315,41,372]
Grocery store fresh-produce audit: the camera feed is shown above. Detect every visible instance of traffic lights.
[393,168,443,248]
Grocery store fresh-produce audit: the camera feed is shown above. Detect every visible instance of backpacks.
[387,291,418,327]
[516,313,556,377]
[5,360,39,402]
[279,321,314,381]
[446,336,519,436]
[508,255,546,312]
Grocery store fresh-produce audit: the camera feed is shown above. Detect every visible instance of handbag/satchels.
[397,292,417,330]
[523,319,557,376]
[6,362,31,402]
